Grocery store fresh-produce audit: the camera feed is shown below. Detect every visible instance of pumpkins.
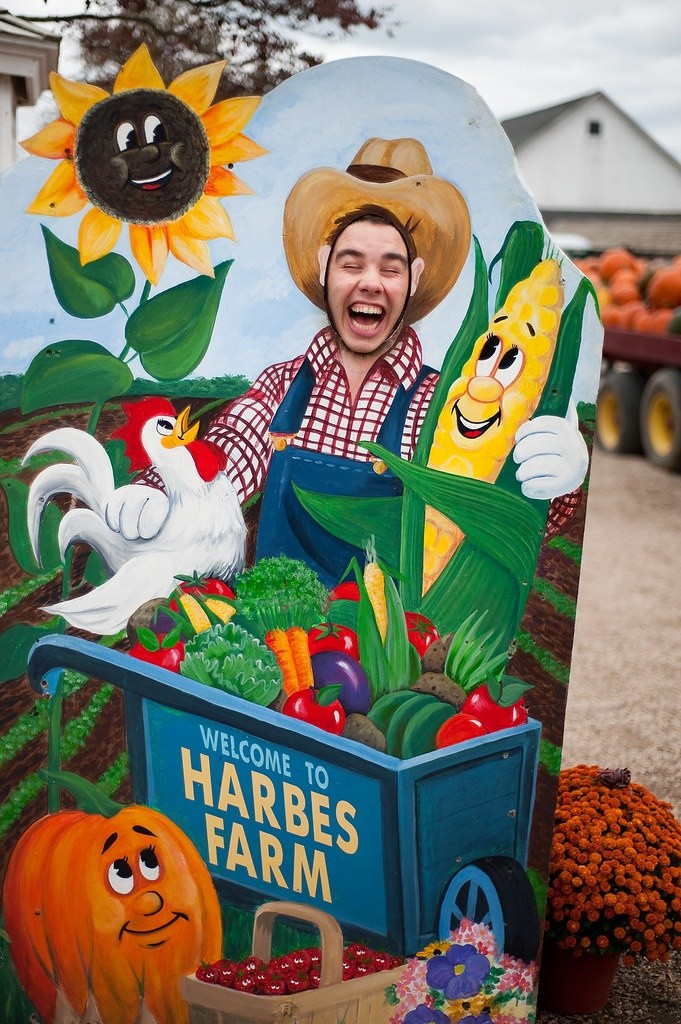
[566,245,681,341]
